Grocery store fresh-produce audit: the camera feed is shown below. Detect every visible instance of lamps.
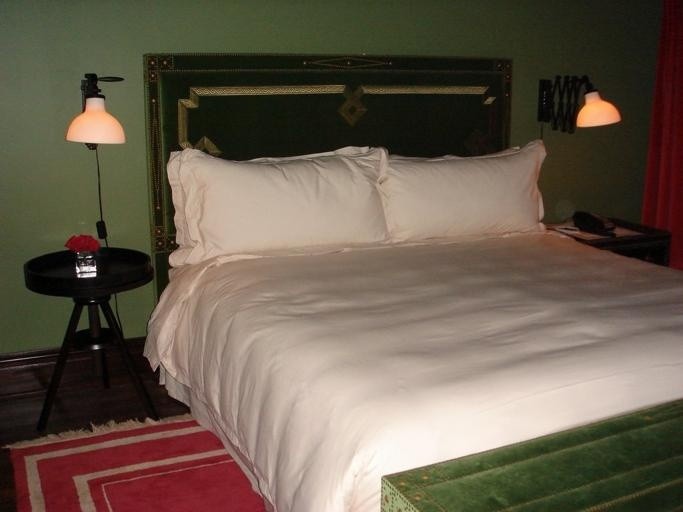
[574,72,622,127]
[64,72,128,151]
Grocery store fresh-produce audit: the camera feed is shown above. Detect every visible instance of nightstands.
[551,216,670,265]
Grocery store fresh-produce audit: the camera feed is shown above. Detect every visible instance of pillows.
[373,140,546,254]
[168,146,388,268]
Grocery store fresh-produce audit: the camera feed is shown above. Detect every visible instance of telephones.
[572,210,616,232]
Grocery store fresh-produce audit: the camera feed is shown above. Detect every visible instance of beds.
[143,51,683,511]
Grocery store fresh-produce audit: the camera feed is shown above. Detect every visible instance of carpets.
[4,414,267,512]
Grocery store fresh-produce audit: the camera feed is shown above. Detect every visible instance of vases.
[75,252,98,279]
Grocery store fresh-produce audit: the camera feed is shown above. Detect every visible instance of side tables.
[21,246,157,435]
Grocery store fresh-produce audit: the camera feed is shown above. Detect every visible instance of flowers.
[64,234,100,256]
